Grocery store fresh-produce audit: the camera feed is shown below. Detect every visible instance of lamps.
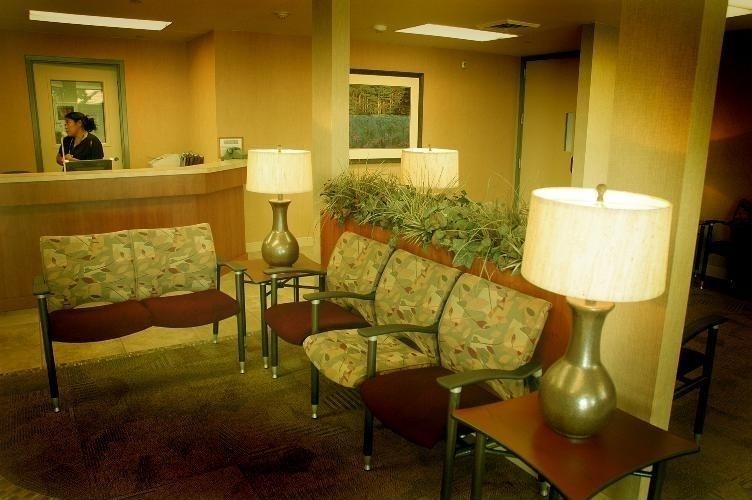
[245,143,314,269]
[521,182,675,442]
[398,144,461,193]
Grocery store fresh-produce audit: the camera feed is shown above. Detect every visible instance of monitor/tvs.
[65,160,112,172]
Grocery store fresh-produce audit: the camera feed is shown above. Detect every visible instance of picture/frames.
[218,136,245,159]
[347,66,424,165]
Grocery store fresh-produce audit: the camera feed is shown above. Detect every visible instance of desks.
[671,304,728,447]
[453,392,704,500]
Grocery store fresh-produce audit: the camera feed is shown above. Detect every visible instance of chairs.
[265,230,551,499]
[35,223,248,414]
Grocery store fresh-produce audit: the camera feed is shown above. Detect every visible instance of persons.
[56,111,104,166]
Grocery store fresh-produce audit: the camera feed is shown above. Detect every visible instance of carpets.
[0,284,752,500]
